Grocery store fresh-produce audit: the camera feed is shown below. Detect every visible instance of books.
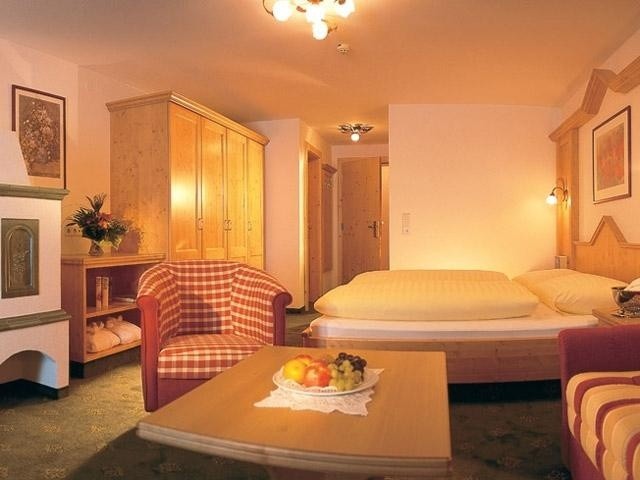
[95,275,110,308]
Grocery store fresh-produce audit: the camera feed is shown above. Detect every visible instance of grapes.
[329,352,366,392]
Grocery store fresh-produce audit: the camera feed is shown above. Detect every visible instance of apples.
[307,361,327,371]
[293,355,313,366]
[283,361,307,385]
[303,366,330,387]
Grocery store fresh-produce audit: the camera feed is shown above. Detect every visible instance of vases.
[88,240,104,258]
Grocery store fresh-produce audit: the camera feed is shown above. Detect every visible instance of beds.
[302,216,640,385]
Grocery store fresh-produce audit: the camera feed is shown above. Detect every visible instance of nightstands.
[592,306,640,328]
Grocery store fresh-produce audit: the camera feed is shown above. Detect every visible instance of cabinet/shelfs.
[61,254,165,378]
[106,92,270,272]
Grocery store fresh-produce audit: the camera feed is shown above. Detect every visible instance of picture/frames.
[592,105,632,204]
[12,84,66,190]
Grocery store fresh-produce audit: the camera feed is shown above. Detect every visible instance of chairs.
[559,324,640,480]
[136,261,293,412]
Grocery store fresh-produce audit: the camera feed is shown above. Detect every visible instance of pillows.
[512,268,630,317]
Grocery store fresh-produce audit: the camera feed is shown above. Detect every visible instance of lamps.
[545,186,568,206]
[337,124,373,142]
[263,0,355,41]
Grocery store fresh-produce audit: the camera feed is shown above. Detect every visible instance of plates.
[272,368,379,396]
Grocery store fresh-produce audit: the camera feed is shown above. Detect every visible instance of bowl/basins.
[610,284,640,316]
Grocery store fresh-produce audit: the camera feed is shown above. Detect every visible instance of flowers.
[65,194,129,249]
